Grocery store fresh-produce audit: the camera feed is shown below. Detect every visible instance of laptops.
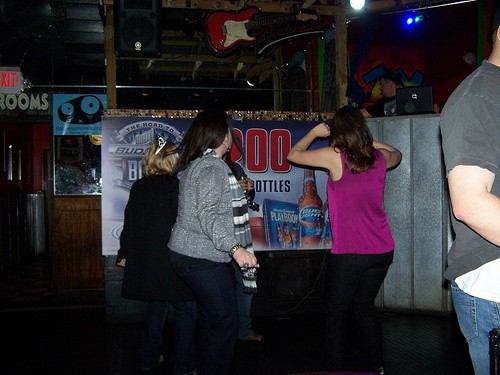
[396,86,434,115]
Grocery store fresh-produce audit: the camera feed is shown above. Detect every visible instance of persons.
[116,137,219,370]
[287,104,402,375]
[437,8,500,375]
[359,71,440,118]
[221,146,264,342]
[167,108,261,375]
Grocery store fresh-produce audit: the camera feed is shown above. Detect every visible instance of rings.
[243,263,249,268]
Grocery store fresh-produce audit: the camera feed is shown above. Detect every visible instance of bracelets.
[230,244,242,256]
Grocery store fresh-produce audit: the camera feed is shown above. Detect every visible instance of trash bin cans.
[16,191,46,257]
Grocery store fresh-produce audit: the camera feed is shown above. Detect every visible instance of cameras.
[488,328,500,375]
[241,264,260,293]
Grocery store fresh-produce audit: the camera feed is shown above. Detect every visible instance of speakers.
[113,0,162,58]
